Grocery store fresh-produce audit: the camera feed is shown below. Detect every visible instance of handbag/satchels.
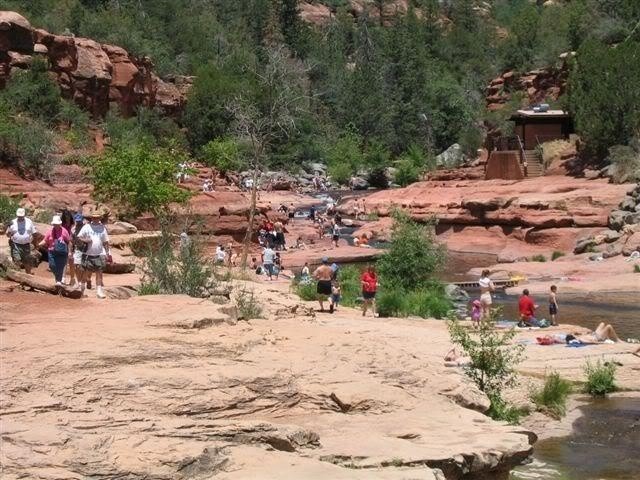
[75,239,88,252]
[54,239,67,254]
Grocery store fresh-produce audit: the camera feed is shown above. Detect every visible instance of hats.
[473,300,481,307]
[321,257,328,265]
[49,215,63,226]
[75,214,82,223]
[91,210,101,218]
[15,208,27,219]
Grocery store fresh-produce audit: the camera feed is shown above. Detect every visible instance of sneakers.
[330,304,334,313]
[54,280,91,293]
[96,288,106,299]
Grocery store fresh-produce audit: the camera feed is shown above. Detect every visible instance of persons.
[177,161,188,184]
[478,270,495,329]
[180,229,189,249]
[360,264,378,318]
[5,208,113,298]
[203,180,212,191]
[217,246,225,266]
[309,198,374,248]
[518,289,540,327]
[245,178,253,194]
[288,204,296,226]
[249,218,285,282]
[302,256,342,314]
[549,285,559,325]
[565,322,622,344]
[377,229,385,242]
[472,300,480,319]
[216,244,222,256]
[278,203,286,214]
